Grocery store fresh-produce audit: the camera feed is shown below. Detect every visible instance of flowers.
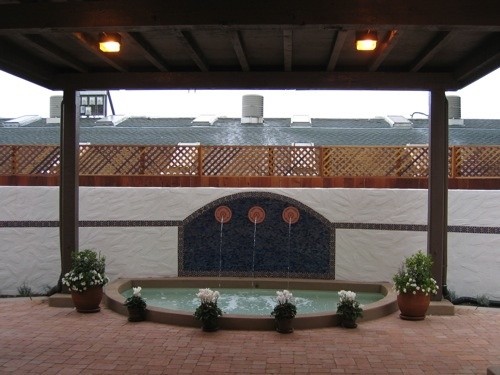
[335,289,365,320]
[192,287,224,321]
[122,285,147,310]
[390,248,440,296]
[60,248,111,293]
[269,289,297,322]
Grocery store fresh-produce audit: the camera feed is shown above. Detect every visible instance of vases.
[275,319,295,334]
[396,291,432,321]
[339,317,357,330]
[201,318,220,333]
[127,308,149,323]
[71,286,104,313]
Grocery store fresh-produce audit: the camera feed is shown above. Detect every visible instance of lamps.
[354,29,378,51]
[96,31,123,53]
